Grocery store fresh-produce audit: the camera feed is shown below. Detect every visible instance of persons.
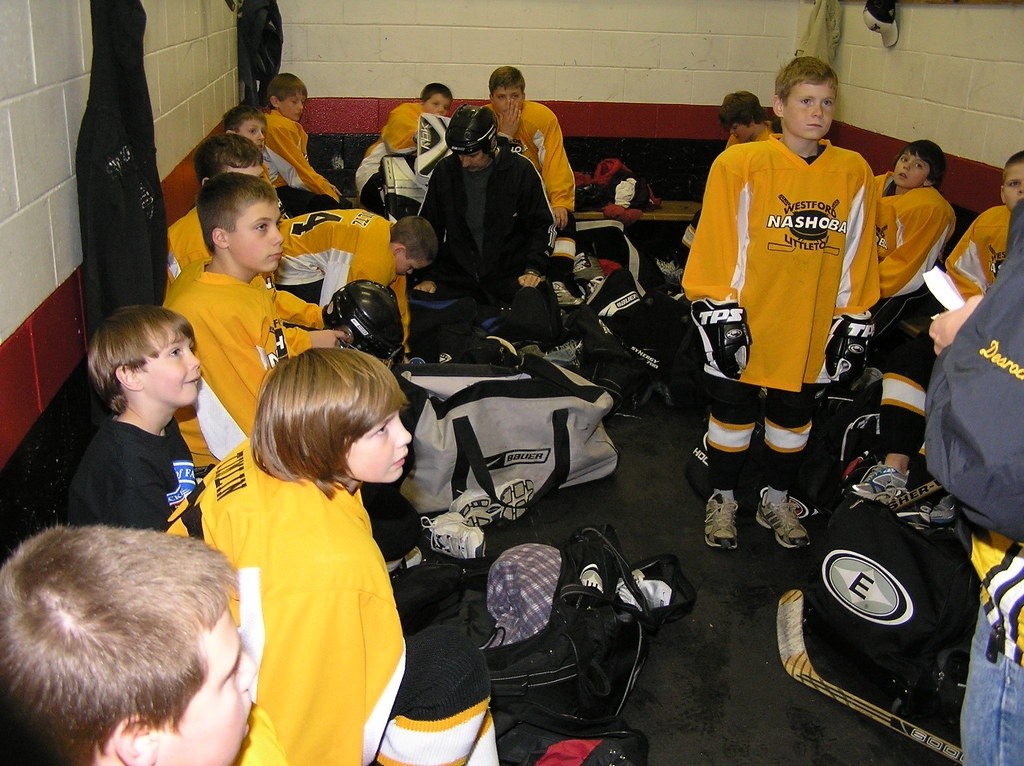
[682,56,879,550]
[168,346,500,766]
[0,522,255,766]
[924,198,1024,766]
[655,92,775,286]
[814,140,1024,525]
[162,172,422,578]
[159,66,584,366]
[53,303,199,533]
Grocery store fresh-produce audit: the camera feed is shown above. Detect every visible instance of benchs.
[0,352,115,567]
[309,131,727,270]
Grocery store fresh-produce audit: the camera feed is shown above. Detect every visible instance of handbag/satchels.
[480,523,652,766]
[388,220,696,525]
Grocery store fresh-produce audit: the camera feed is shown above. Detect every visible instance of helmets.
[444,103,496,155]
[322,279,405,358]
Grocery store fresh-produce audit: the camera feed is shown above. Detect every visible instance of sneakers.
[705,492,739,550]
[894,493,956,522]
[756,487,810,548]
[846,461,910,506]
[653,253,684,286]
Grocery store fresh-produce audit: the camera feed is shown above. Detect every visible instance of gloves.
[689,296,755,380]
[823,314,875,388]
